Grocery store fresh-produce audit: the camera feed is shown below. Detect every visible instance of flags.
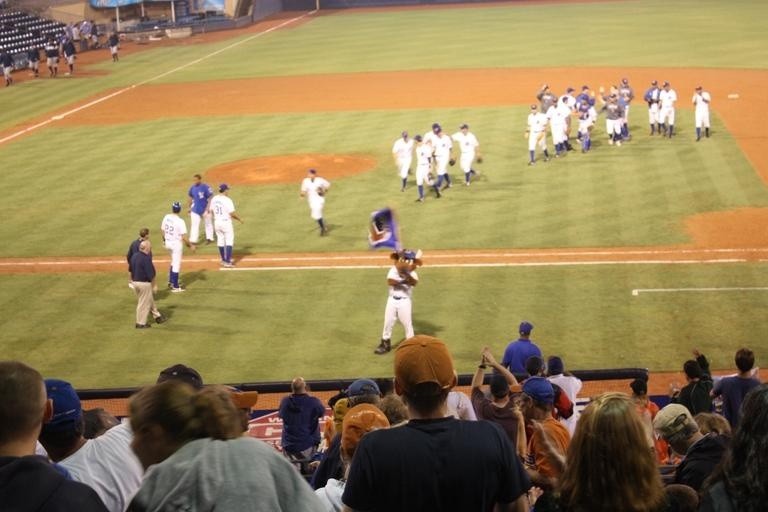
[367,208,398,250]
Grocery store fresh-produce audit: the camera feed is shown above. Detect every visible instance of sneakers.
[168,283,186,292]
[221,261,235,267]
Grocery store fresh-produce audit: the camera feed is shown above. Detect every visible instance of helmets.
[172,202,181,211]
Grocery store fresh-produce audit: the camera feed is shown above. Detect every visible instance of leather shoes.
[155,317,168,324]
[136,324,151,328]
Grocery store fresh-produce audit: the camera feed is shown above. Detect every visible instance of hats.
[630,380,647,395]
[548,356,564,374]
[491,375,506,393]
[218,184,230,191]
[519,322,533,333]
[652,403,693,438]
[210,383,258,408]
[43,380,82,426]
[509,376,555,402]
[341,379,390,457]
[395,334,454,395]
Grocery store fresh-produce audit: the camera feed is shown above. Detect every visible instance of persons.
[126,363,410,512]
[90,24,99,49]
[0,361,146,512]
[708,346,760,430]
[62,35,77,78]
[79,29,86,51]
[341,334,534,512]
[298,169,331,238]
[443,324,734,512]
[692,86,711,141]
[373,249,423,355]
[184,174,215,245]
[699,383,767,512]
[206,183,244,268]
[0,49,16,87]
[127,239,168,329]
[27,42,40,79]
[126,228,153,302]
[44,37,60,79]
[110,32,118,63]
[390,123,484,204]
[522,78,677,165]
[159,200,197,293]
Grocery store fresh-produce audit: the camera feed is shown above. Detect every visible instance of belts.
[393,297,406,300]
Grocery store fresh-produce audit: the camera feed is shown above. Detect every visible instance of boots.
[375,339,391,354]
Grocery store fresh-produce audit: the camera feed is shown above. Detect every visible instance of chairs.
[0,6,82,60]
